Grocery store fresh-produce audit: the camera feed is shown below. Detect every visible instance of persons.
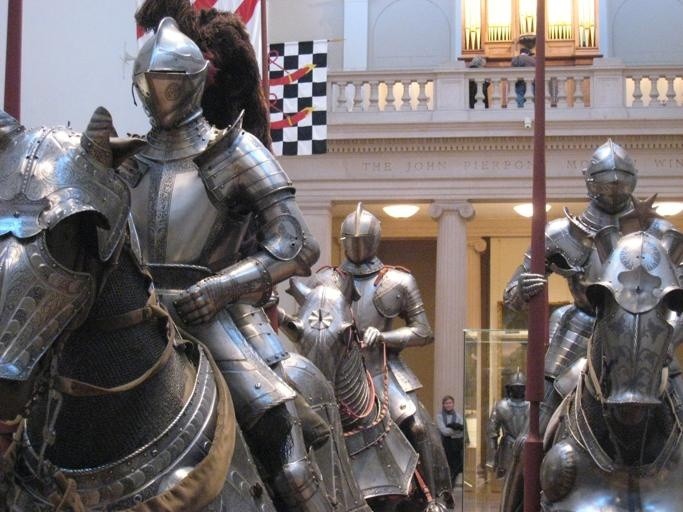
[0,16,683,512]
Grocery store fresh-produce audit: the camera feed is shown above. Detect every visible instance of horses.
[0,105,374,512]
[540,224,683,512]
[279,269,456,512]
